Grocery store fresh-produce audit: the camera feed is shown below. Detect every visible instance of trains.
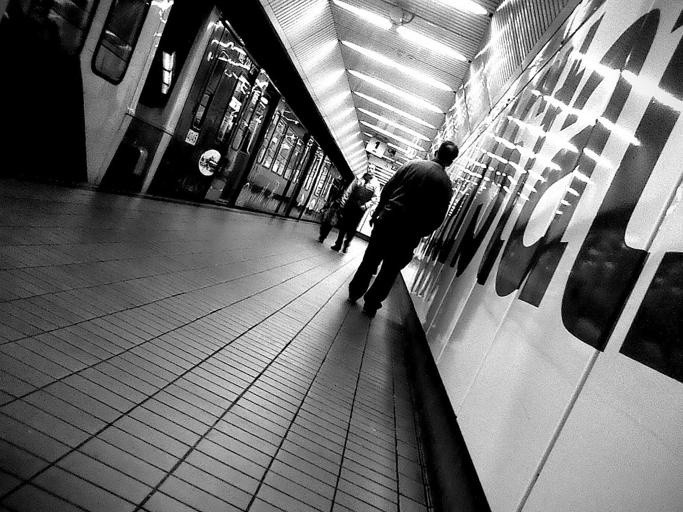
[5,0,338,222]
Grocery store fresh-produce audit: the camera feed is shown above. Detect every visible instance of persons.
[348,142,457,315]
[317,197,342,242]
[331,163,381,251]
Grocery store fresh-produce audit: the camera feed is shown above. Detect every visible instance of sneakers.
[331,245,341,252]
[361,304,377,319]
[343,241,349,253]
[347,298,357,303]
